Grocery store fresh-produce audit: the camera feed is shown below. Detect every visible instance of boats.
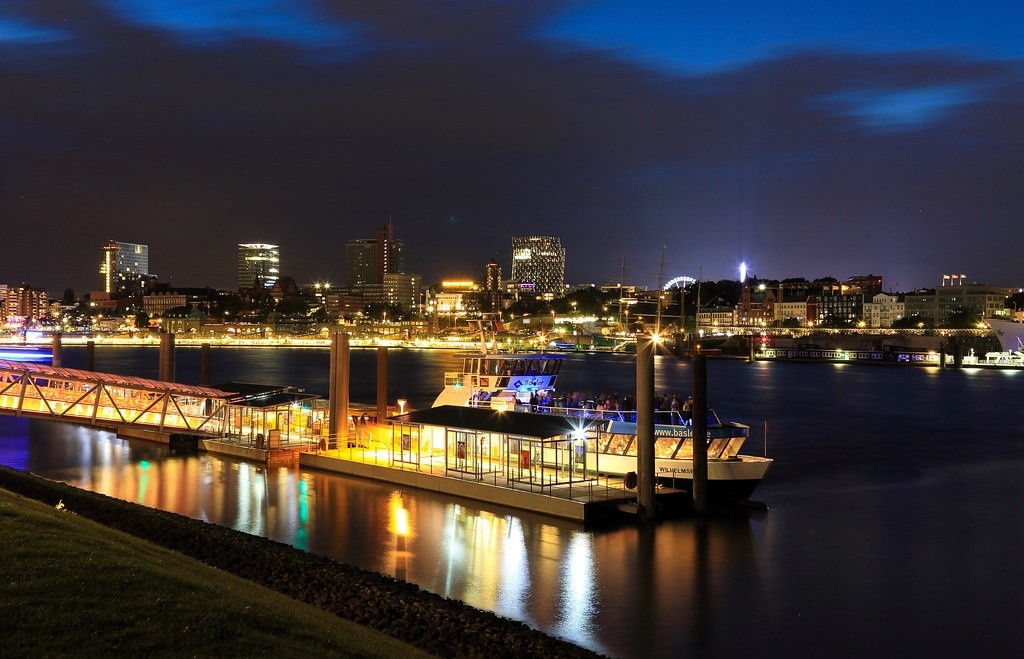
[420,318,774,520]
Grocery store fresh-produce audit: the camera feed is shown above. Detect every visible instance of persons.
[525,389,692,425]
